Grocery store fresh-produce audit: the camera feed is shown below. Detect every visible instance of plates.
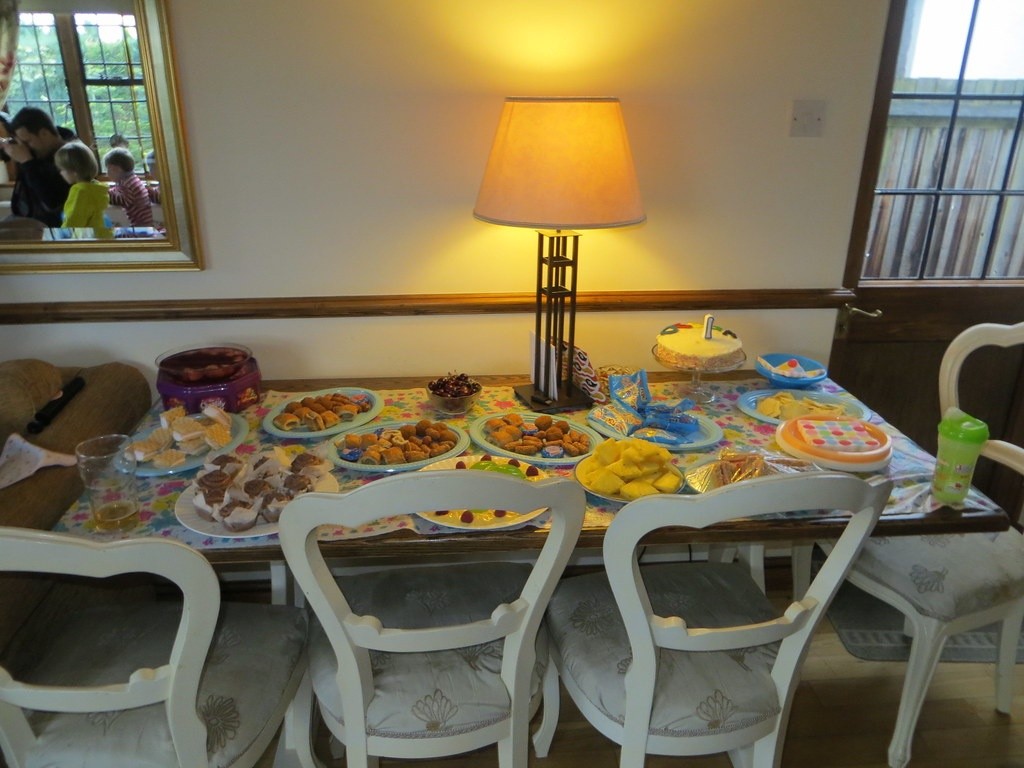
[683,455,714,494]
[174,471,339,539]
[736,389,872,426]
[327,420,471,474]
[413,455,549,531]
[586,405,725,452]
[775,417,894,472]
[263,387,385,438]
[469,410,605,466]
[112,411,249,477]
[573,455,686,503]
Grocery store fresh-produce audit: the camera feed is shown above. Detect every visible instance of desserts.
[189,447,336,533]
[124,403,233,469]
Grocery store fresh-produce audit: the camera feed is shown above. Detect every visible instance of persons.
[0,107,115,240]
[105,134,172,243]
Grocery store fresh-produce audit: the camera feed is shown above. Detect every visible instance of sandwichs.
[713,452,779,489]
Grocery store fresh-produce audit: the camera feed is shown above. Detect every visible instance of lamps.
[473,98,646,414]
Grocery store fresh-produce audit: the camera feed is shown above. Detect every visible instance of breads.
[273,404,358,429]
[346,430,430,465]
[484,412,524,448]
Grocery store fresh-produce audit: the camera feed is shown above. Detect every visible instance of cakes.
[656,313,743,368]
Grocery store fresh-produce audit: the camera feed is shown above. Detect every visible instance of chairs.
[1,524,309,768]
[531,472,896,767]
[942,322,1024,530]
[280,470,586,767]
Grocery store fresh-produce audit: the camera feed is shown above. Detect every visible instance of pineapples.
[585,436,683,501]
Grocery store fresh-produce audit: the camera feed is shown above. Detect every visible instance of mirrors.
[0,0,205,273]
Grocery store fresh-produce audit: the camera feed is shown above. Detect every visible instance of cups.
[931,406,989,506]
[75,434,141,536]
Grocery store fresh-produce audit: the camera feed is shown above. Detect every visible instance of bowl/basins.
[155,341,253,384]
[754,351,827,390]
[426,379,483,415]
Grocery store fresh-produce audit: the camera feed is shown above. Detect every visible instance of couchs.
[0,361,149,673]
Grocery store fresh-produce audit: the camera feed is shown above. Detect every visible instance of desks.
[47,368,1008,613]
